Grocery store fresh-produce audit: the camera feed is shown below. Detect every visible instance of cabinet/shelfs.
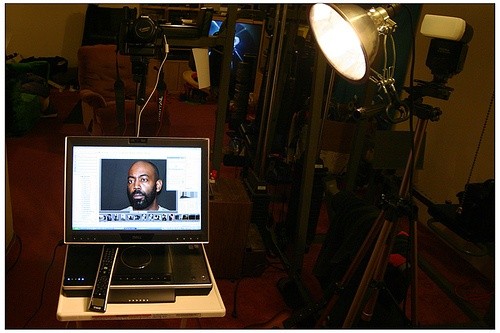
[238,4,422,330]
[146,59,191,95]
[138,4,202,24]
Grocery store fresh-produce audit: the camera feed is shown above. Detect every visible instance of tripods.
[317,118,421,329]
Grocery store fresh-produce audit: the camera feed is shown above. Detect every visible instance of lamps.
[309,3,474,130]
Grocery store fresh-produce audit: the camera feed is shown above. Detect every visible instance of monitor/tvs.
[208,16,263,56]
[62,134,212,245]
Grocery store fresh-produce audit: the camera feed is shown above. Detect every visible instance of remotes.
[88,246,119,312]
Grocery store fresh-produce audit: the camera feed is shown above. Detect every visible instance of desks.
[56,244,226,328]
[208,176,254,280]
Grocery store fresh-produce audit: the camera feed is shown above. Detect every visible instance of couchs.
[182,67,211,103]
[77,44,169,137]
[5,61,51,137]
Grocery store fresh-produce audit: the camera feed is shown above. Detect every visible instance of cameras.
[116,15,165,55]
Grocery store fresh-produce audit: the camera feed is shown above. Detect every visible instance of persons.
[121,160,170,212]
[210,20,241,96]
